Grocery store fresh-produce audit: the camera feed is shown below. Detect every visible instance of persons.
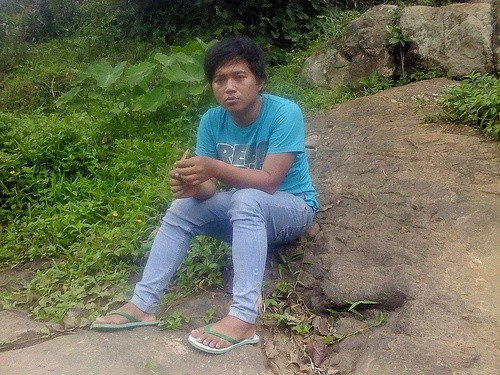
[91,36,319,354]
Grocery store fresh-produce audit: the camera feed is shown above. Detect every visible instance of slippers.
[187,323,260,355]
[92,309,163,328]
